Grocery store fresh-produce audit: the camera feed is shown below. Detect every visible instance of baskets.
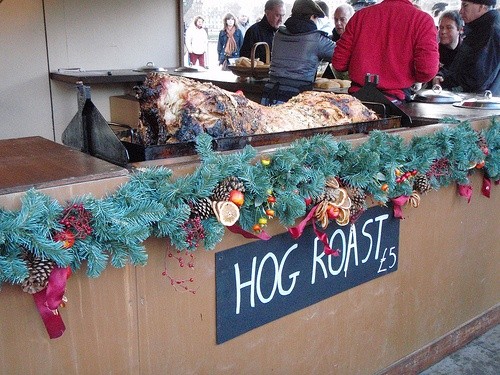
[228,42,270,78]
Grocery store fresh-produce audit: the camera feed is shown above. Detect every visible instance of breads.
[315,77,351,89]
[235,57,264,67]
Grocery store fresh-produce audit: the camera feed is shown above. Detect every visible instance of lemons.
[326,177,351,226]
[467,161,477,169]
[212,201,240,227]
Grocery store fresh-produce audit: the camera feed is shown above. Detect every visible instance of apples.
[479,146,489,153]
[229,190,244,206]
[304,197,311,205]
[55,230,75,247]
[326,204,339,219]
[476,160,485,168]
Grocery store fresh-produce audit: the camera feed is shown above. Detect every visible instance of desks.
[0,65,500,375]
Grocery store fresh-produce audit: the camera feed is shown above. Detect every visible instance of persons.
[217,13,243,71]
[238,0,500,105]
[185,16,208,68]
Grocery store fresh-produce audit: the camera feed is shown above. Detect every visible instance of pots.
[452,89,500,110]
[414,83,462,104]
[174,62,208,72]
[133,61,168,72]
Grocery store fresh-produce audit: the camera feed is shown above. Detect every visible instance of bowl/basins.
[228,65,269,78]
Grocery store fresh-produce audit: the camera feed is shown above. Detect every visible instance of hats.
[293,0,325,18]
[315,0,329,18]
[460,0,496,5]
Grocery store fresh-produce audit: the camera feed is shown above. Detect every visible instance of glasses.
[461,2,471,8]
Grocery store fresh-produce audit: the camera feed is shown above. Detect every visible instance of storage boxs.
[111,95,141,131]
